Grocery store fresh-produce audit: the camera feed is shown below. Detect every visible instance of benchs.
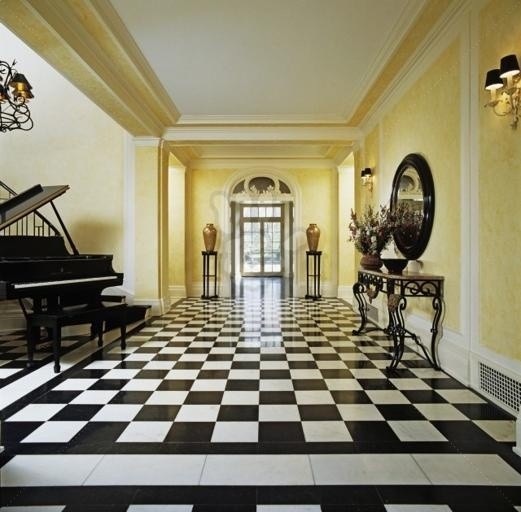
[25,301,128,374]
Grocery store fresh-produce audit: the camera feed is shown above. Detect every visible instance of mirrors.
[389,153,436,259]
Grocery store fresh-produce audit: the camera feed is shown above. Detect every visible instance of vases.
[305,224,321,252]
[359,252,383,271]
[202,223,217,252]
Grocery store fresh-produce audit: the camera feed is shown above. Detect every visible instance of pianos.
[0,185,124,338]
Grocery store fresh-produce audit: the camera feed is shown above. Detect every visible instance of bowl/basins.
[382,259,409,274]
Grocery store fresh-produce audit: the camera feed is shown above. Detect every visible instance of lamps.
[484,53,521,132]
[0,57,35,134]
[360,167,373,193]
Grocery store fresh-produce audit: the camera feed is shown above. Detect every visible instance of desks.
[201,250,219,300]
[352,269,445,373]
[305,250,322,302]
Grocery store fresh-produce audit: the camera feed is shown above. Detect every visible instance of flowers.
[396,201,424,248]
[346,203,399,257]
